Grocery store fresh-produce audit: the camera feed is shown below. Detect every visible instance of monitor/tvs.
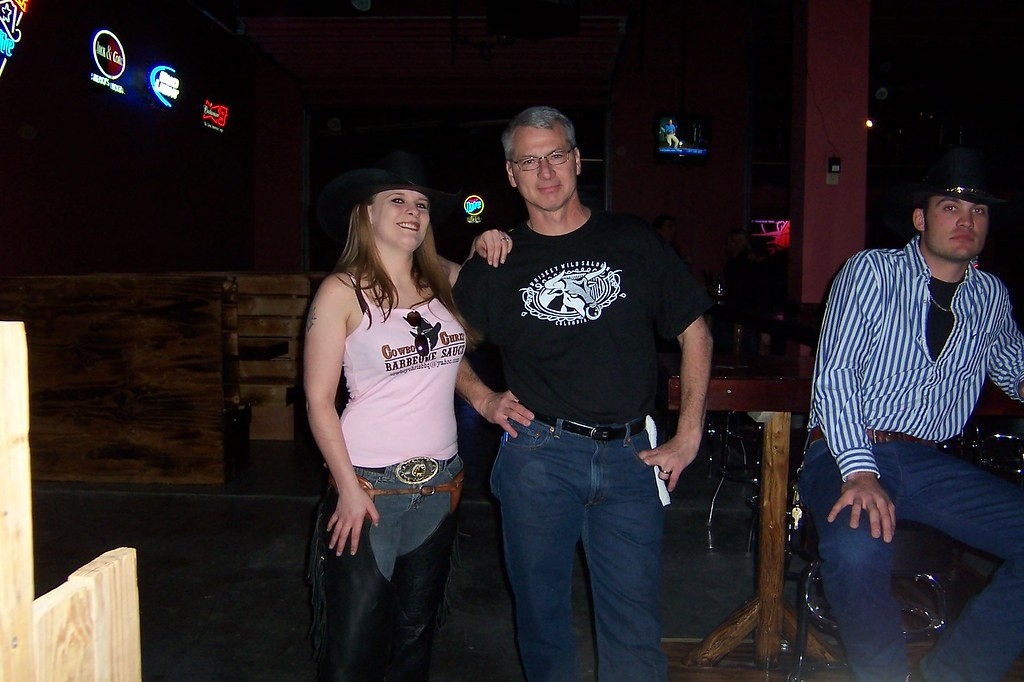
[652,109,713,166]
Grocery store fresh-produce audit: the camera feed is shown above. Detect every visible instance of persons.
[797,165,1024,679]
[303,147,514,680]
[435,104,716,682]
[655,210,682,258]
[717,219,793,351]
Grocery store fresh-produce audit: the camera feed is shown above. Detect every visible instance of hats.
[339,152,438,205]
[922,148,1007,207]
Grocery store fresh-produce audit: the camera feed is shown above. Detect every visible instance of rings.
[503,236,508,239]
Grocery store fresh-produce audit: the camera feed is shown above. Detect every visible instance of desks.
[675,358,834,670]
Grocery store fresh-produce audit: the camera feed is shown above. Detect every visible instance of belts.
[809,425,939,447]
[532,413,657,441]
[353,452,458,477]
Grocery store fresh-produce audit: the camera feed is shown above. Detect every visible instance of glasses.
[406,309,429,358]
[508,144,578,172]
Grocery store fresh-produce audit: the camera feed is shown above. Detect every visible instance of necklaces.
[926,284,953,313]
